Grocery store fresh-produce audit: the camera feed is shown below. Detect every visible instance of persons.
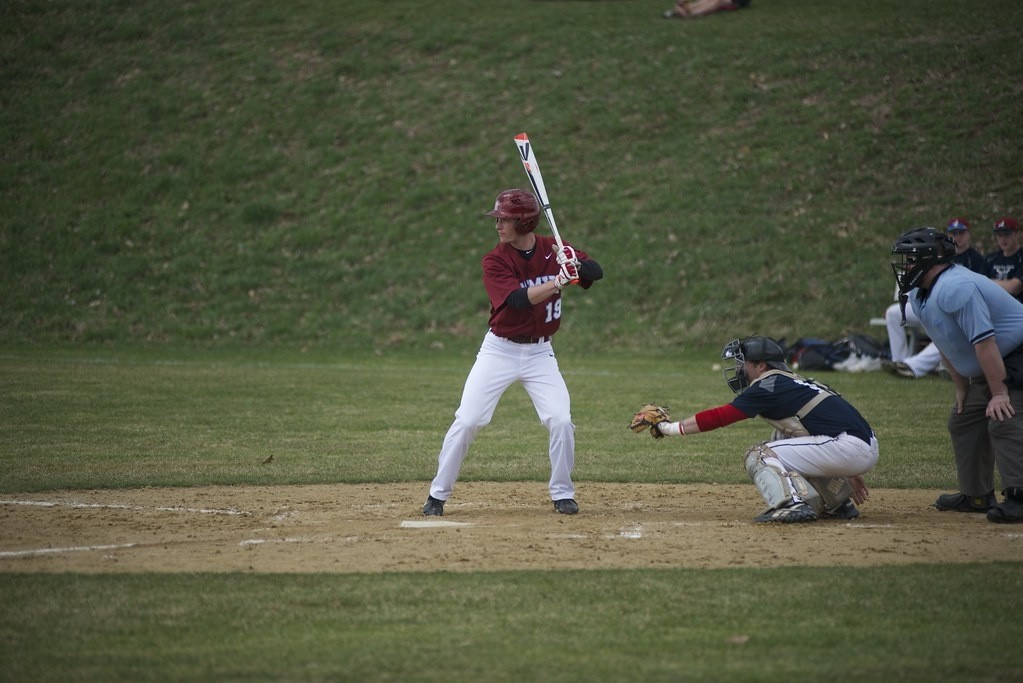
[887,217,1023,379]
[888,227,1023,524]
[885,218,986,378]
[627,332,879,523]
[422,189,604,517]
[674,0,751,19]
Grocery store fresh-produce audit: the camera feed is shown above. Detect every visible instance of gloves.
[552,245,578,264]
[554,262,579,289]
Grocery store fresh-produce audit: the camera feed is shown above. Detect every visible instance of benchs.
[870,271,921,356]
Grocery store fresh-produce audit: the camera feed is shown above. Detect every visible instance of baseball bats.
[513,130,579,287]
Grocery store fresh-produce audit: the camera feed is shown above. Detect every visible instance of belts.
[510,337,551,343]
[846,430,872,446]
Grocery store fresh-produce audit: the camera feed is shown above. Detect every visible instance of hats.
[947,219,971,230]
[738,337,793,373]
[993,219,1021,231]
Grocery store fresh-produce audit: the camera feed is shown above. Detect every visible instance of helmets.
[891,226,952,294]
[485,190,539,235]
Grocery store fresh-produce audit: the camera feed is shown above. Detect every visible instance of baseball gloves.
[630,404,672,439]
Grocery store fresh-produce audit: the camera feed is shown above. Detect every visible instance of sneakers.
[987,489,1023,524]
[754,503,817,522]
[822,502,859,518]
[422,501,445,517]
[935,493,997,512]
[554,500,579,514]
[882,361,914,378]
